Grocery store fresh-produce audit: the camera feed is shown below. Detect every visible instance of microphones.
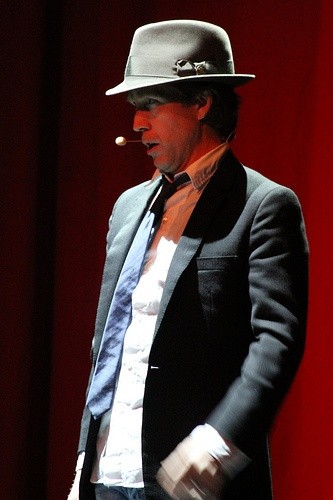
[116,136,143,146]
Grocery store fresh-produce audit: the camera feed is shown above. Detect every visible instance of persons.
[66,20,310,500]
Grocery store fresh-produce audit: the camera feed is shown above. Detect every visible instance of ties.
[85,173,191,421]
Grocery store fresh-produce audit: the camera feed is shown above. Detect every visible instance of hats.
[105,19,255,96]
